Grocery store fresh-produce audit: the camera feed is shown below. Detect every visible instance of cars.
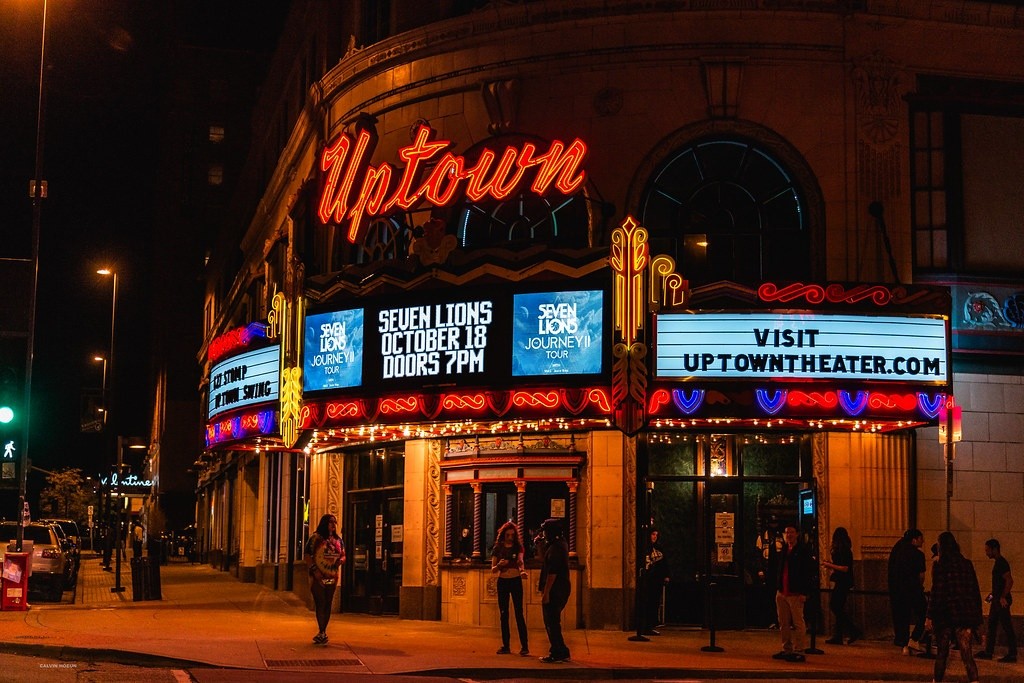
[0,517,82,604]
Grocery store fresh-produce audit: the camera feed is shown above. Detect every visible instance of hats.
[541,519,561,531]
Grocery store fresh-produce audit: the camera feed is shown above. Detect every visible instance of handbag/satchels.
[952,601,983,626]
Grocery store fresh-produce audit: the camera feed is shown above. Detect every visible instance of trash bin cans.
[131,556,161,601]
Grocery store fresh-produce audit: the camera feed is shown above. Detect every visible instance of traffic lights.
[0,365,23,462]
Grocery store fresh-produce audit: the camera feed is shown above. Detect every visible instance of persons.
[973,539,1018,663]
[637,528,669,634]
[771,524,815,662]
[131,520,143,556]
[305,514,345,644]
[918,532,982,683]
[536,519,570,662]
[823,528,863,644]
[889,528,927,655]
[493,523,530,656]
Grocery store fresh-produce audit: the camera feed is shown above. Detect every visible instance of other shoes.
[974,651,993,660]
[908,639,926,652]
[496,647,510,654]
[826,635,843,644]
[539,651,563,663]
[519,648,529,657]
[785,653,805,662]
[997,653,1018,663]
[848,631,864,645]
[772,651,785,659]
[566,646,571,662]
[313,633,329,644]
[902,646,909,655]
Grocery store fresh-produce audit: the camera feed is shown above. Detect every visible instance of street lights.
[96,268,117,572]
[94,355,108,427]
